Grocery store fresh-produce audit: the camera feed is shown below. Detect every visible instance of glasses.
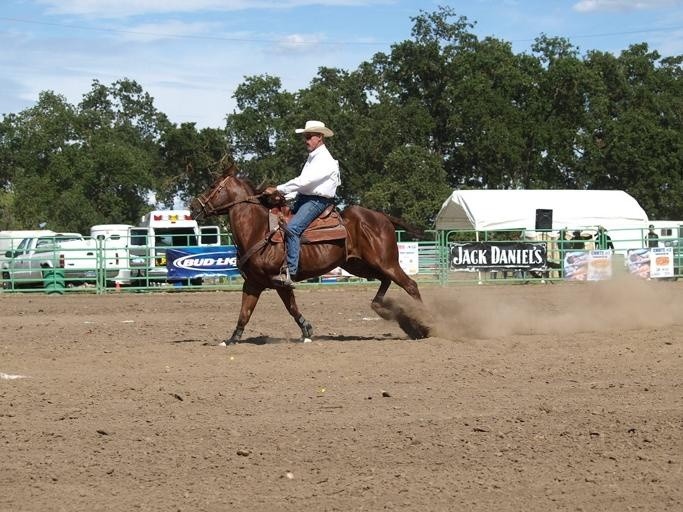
[304,134,321,139]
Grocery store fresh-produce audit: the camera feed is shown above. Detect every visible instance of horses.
[190,163,433,346]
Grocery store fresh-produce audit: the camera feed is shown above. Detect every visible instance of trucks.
[0,208,223,293]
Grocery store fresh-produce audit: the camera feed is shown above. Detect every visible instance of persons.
[645,225,658,247]
[557,230,571,248]
[262,120,342,287]
[595,225,614,249]
[570,230,597,248]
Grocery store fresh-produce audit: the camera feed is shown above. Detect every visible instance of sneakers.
[271,274,296,290]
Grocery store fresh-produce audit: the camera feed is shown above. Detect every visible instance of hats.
[294,120,334,137]
